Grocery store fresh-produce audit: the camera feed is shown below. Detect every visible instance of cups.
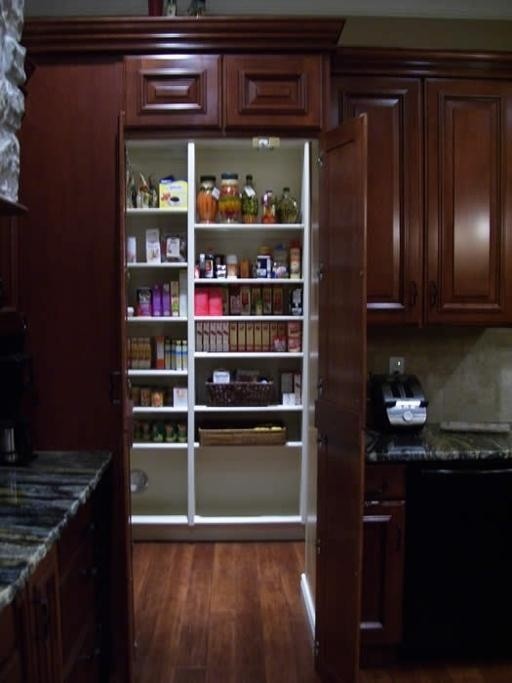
[147,0,163,17]
[131,384,168,407]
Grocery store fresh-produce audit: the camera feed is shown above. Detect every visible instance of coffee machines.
[1,329,41,467]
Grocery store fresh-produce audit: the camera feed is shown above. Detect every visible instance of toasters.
[365,373,429,434]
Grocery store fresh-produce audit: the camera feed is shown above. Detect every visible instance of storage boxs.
[157,176,190,208]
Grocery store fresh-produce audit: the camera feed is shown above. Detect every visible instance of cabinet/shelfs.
[363,457,406,668]
[119,12,344,134]
[56,479,114,683]
[1,545,66,681]
[331,41,509,330]
[124,134,314,545]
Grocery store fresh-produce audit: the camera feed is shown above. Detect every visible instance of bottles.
[165,0,177,17]
[286,320,301,352]
[196,170,300,225]
[193,238,301,278]
